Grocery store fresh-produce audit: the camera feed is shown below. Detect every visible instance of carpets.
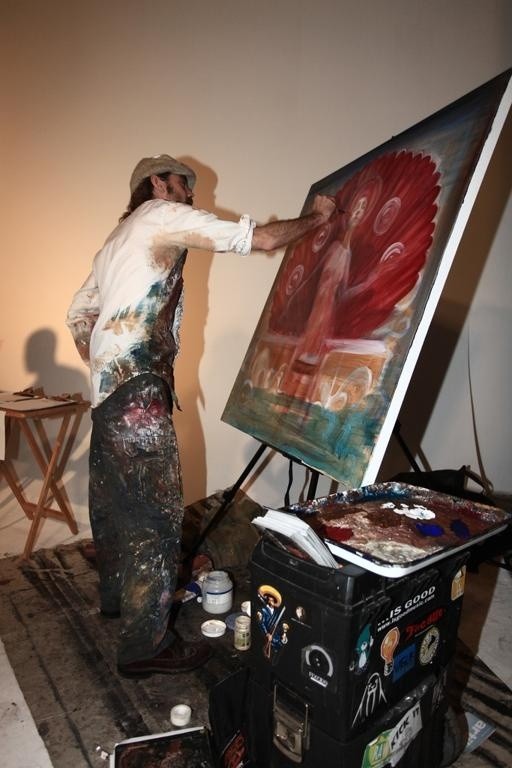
[1,484,511,767]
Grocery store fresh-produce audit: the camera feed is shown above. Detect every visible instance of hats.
[129,154,197,196]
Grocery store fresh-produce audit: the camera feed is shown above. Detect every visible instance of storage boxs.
[245,670,444,768]
[238,536,469,741]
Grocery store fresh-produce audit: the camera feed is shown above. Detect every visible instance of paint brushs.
[6,397,39,401]
[335,208,344,213]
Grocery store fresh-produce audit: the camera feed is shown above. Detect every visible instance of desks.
[0,389,91,561]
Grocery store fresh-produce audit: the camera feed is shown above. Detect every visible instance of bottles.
[232,615,252,651]
[200,570,234,614]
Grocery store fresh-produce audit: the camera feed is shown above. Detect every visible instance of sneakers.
[117,637,213,679]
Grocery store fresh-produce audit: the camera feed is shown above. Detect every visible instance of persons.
[65,158,337,679]
[283,194,400,401]
